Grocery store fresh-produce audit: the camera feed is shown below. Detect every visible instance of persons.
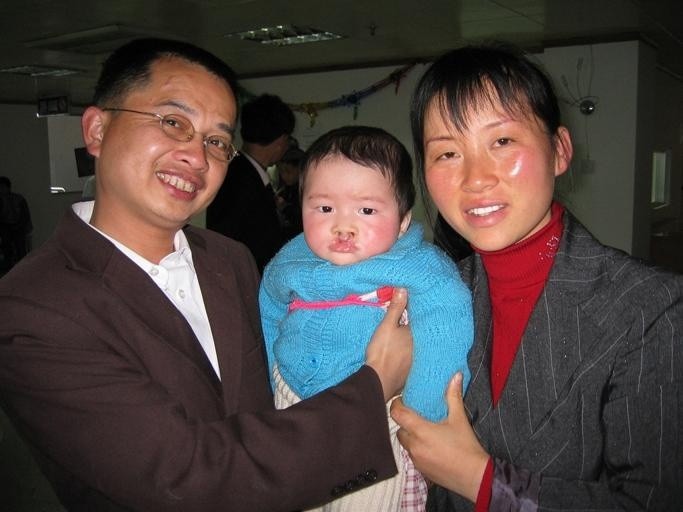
[389,42,683,511]
[273,148,305,247]
[259,124,474,512]
[0,35,413,511]
[204,93,297,276]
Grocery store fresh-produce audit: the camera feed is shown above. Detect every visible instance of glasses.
[101,107,240,164]
